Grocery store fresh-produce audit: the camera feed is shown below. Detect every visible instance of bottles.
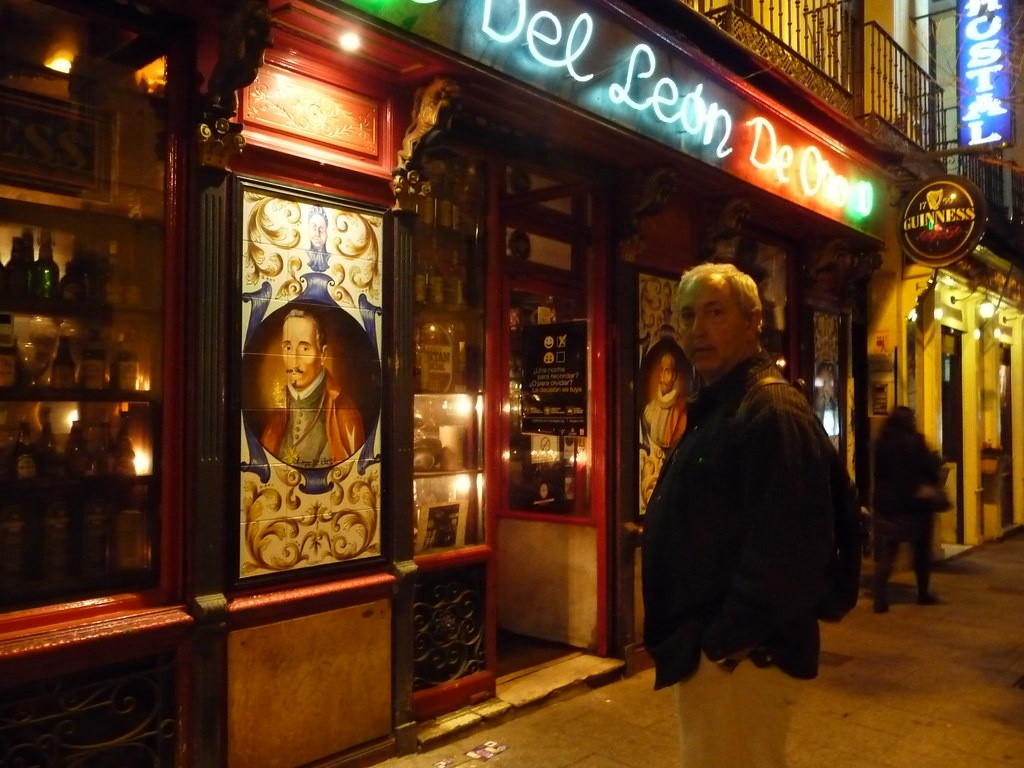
[0,227,137,609]
[412,167,466,306]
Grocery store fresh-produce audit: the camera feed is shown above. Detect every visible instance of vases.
[980,457,1012,474]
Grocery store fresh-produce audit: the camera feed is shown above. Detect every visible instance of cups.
[438,425,464,472]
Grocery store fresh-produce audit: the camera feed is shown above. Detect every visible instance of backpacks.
[732,377,862,621]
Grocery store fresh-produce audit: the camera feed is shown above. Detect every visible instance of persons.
[639,262,861,767]
[872,405,948,614]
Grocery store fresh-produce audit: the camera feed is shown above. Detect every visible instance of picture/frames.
[0,83,121,202]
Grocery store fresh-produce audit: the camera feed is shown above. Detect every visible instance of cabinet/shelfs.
[415,220,487,553]
[0,198,167,617]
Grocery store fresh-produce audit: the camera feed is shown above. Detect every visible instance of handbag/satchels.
[911,483,951,512]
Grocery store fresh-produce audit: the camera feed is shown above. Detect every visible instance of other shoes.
[873,599,889,613]
[917,596,936,606]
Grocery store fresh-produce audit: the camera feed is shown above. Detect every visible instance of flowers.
[982,439,1005,456]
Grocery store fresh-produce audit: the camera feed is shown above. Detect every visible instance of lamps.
[951,281,994,319]
